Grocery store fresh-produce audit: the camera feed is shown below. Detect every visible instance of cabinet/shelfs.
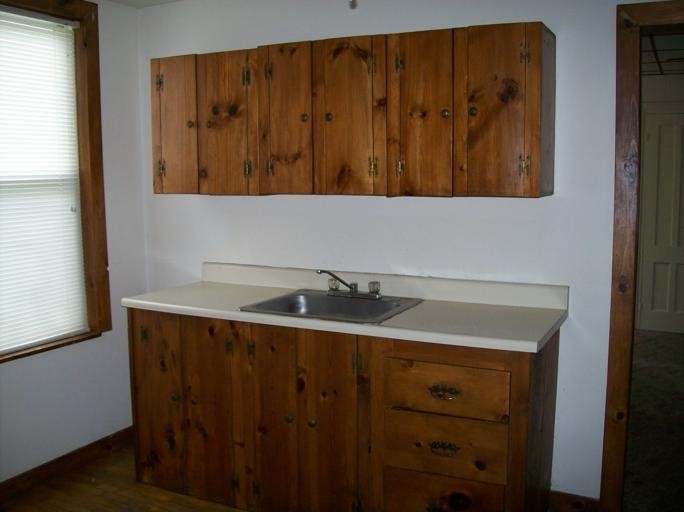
[151,52,248,195]
[387,24,554,195]
[255,36,373,196]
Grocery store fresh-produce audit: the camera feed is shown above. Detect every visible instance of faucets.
[314,267,360,294]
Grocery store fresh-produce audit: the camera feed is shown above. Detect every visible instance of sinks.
[235,287,425,326]
